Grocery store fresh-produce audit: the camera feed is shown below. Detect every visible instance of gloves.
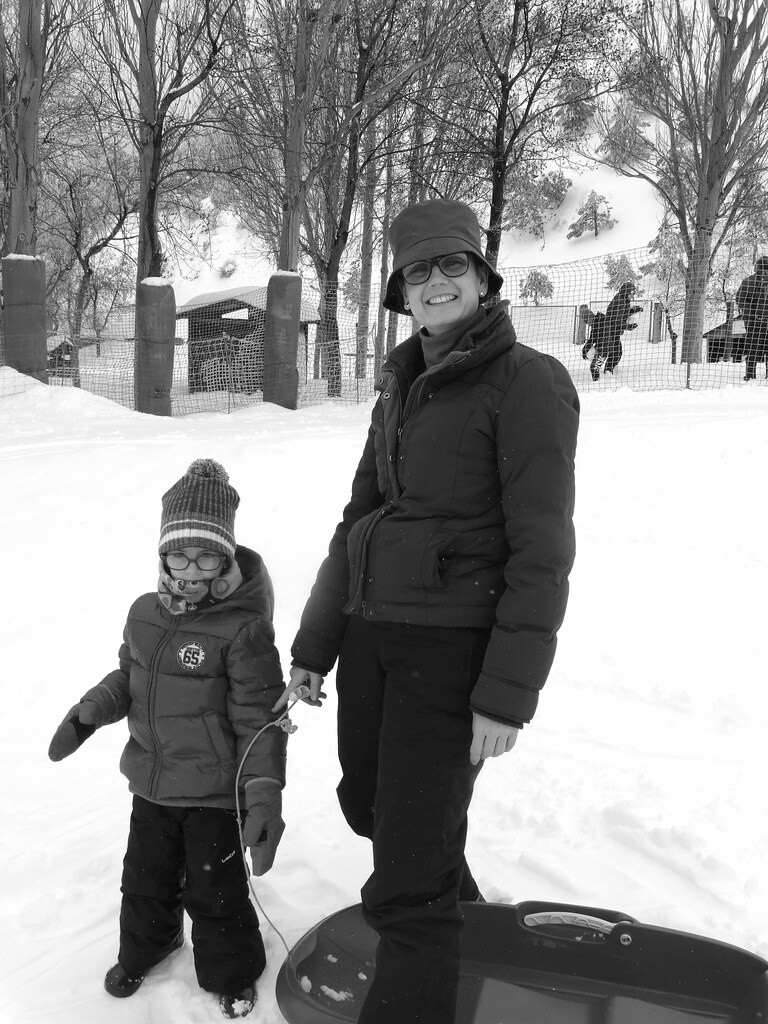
[48,684,119,762]
[243,778,284,877]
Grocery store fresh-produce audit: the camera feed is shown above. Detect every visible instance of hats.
[382,199,503,317]
[158,458,240,577]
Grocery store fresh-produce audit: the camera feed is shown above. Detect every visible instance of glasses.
[401,252,470,286]
[160,552,227,571]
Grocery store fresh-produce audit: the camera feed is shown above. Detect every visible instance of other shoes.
[218,983,257,1020]
[105,963,146,999]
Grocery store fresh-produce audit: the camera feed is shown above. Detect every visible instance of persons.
[735,256,768,381]
[271,198,581,1024]
[580,282,644,382]
[47,457,290,1021]
